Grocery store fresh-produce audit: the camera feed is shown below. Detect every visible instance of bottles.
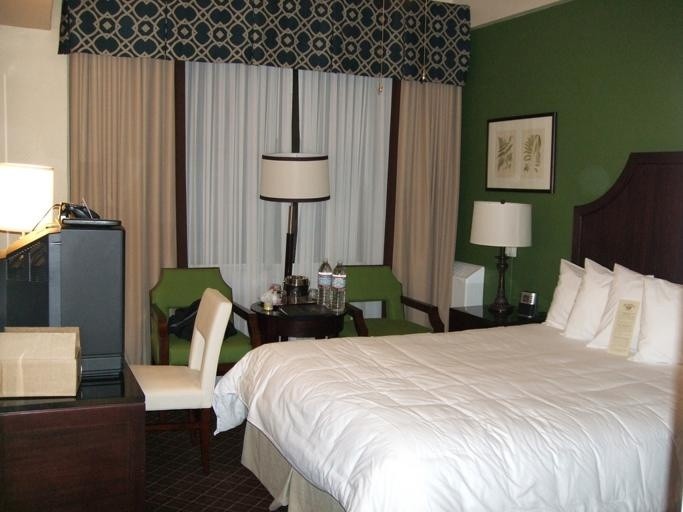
[331,260,346,312]
[317,260,332,306]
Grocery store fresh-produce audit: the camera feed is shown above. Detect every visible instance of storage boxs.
[0,326,84,398]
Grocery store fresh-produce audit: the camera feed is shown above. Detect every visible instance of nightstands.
[448,301,546,334]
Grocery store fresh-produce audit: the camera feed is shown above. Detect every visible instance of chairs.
[151,267,262,424]
[333,265,444,338]
[127,288,234,476]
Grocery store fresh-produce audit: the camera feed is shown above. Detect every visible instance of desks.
[0,356,145,512]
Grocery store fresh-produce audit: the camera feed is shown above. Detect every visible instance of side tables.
[251,293,346,345]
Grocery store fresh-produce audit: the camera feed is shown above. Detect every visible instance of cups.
[309,289,318,301]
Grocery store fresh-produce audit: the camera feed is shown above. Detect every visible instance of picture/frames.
[484,112,556,193]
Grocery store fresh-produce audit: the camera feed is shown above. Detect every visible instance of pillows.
[541,258,683,364]
[167,297,237,343]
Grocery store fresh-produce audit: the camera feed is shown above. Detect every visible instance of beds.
[213,153,683,512]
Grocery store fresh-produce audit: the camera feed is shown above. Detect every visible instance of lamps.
[0,161,54,247]
[470,200,533,316]
[258,153,330,302]
